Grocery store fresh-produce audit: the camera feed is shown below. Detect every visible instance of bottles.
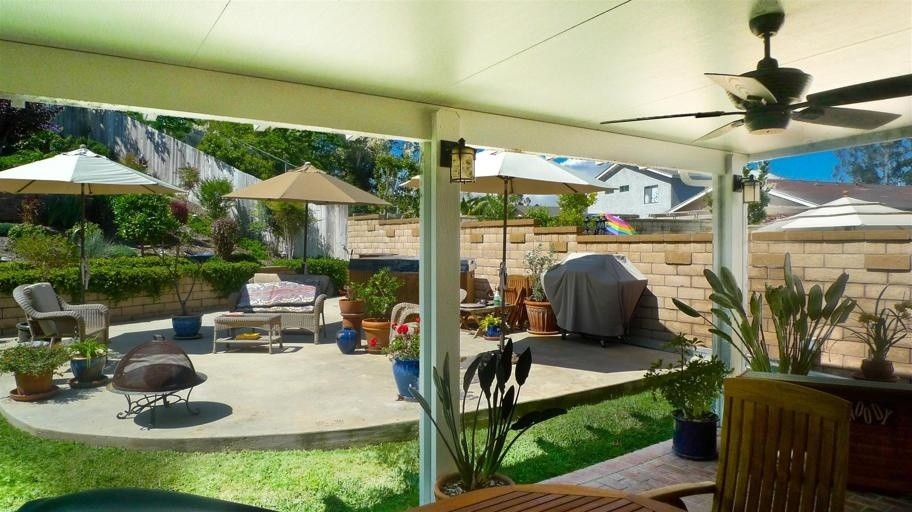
[494,290,501,306]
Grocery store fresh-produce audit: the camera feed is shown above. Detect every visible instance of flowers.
[369,316,421,359]
[817,282,912,362]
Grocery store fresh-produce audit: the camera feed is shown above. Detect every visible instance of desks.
[107,372,208,430]
[460,303,514,339]
[408,483,691,512]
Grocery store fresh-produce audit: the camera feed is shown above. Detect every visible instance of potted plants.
[523,243,559,332]
[481,315,503,338]
[7,222,72,341]
[338,280,364,314]
[643,332,735,461]
[409,338,567,502]
[65,334,111,381]
[112,193,203,337]
[361,267,406,349]
[0,344,72,396]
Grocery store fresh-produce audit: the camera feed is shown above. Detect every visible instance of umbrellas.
[399,152,619,352]
[0,143,190,305]
[597,211,639,236]
[217,161,392,275]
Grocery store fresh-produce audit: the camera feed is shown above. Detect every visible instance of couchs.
[229,273,334,345]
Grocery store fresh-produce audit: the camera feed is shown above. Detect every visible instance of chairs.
[638,377,852,511]
[12,281,110,364]
[389,288,468,341]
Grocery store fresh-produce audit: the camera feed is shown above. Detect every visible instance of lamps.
[440,138,476,185]
[732,174,762,203]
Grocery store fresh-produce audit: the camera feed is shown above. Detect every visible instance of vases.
[861,360,894,380]
[335,326,359,354]
[392,357,419,397]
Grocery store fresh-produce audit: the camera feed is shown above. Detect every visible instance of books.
[224,311,245,316]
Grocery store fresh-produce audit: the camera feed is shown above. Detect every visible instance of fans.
[599,9,912,144]
